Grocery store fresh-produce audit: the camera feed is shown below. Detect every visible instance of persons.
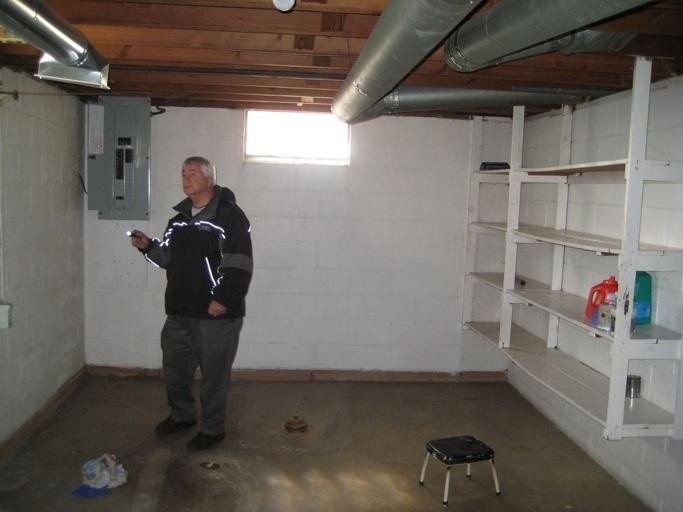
[130,156,254,454]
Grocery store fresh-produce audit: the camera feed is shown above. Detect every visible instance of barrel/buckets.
[627,271,652,324]
[585,276,618,319]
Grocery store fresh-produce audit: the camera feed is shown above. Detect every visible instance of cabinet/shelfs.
[461,56,683,441]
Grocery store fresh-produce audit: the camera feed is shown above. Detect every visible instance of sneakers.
[186,431,225,451]
[154,413,196,434]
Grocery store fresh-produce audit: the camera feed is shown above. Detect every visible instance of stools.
[418,436,502,508]
